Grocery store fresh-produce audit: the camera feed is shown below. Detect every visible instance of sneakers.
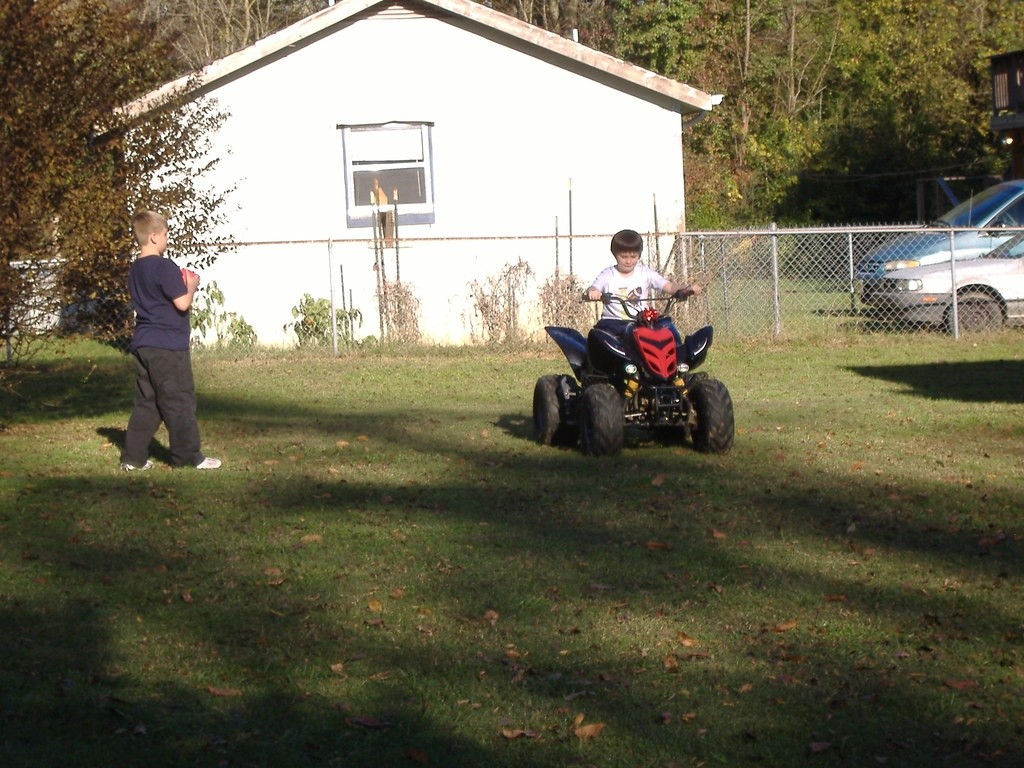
[170,457,222,470]
[119,461,153,471]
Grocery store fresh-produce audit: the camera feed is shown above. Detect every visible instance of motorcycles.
[533,288,735,456]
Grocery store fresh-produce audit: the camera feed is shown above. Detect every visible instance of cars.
[856,180,1024,337]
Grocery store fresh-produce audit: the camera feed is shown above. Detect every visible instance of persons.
[587,229,702,345]
[118,207,221,470]
